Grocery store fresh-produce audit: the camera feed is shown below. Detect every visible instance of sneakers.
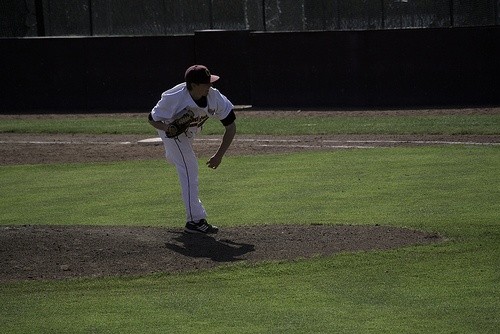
[183,218,219,234]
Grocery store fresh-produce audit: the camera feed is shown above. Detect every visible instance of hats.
[185,65,220,83]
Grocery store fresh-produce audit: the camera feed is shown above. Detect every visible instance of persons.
[148,64,236,235]
